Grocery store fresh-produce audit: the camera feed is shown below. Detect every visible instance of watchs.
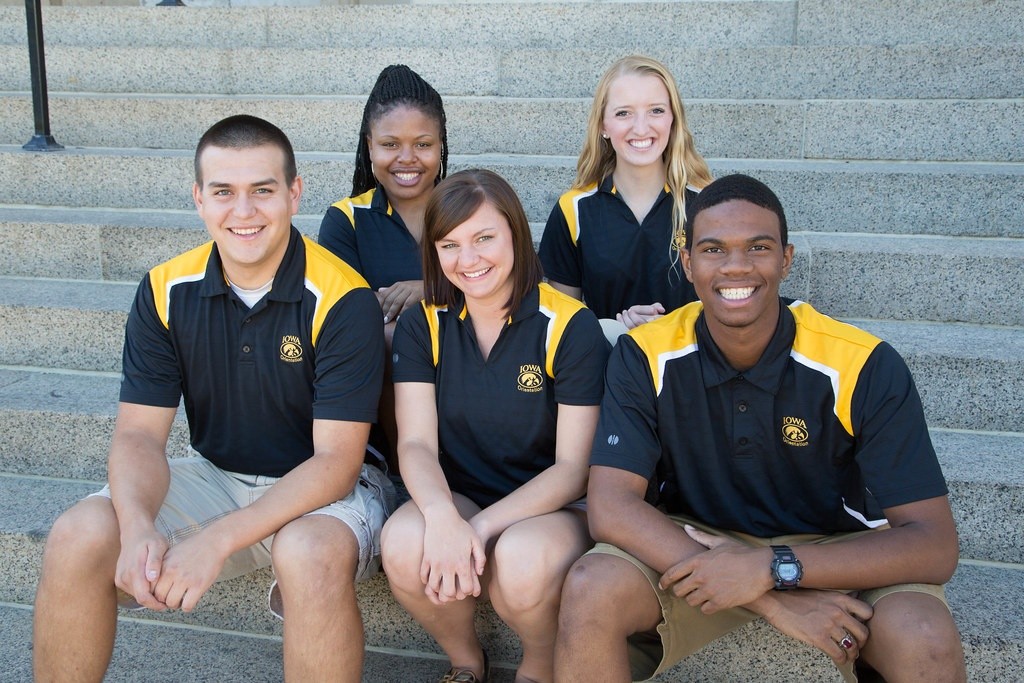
[770,545,804,592]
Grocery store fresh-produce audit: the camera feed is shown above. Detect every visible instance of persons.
[318,62,448,474]
[33,115,397,683]
[539,56,713,347]
[554,175,967,683]
[380,168,610,683]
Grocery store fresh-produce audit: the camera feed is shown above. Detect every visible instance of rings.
[839,633,852,651]
